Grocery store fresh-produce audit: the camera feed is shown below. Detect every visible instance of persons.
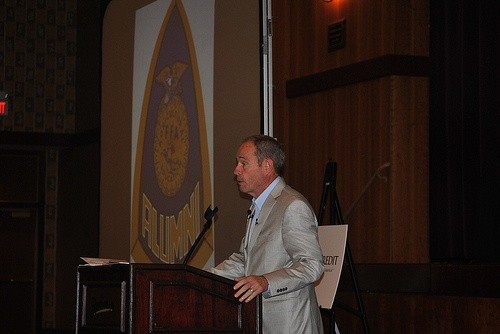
[204,133,326,334]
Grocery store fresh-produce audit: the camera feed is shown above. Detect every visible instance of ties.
[246,202,256,255]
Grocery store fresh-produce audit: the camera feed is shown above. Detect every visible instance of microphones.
[247,209,254,219]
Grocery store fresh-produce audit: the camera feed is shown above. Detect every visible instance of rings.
[249,288,254,293]
